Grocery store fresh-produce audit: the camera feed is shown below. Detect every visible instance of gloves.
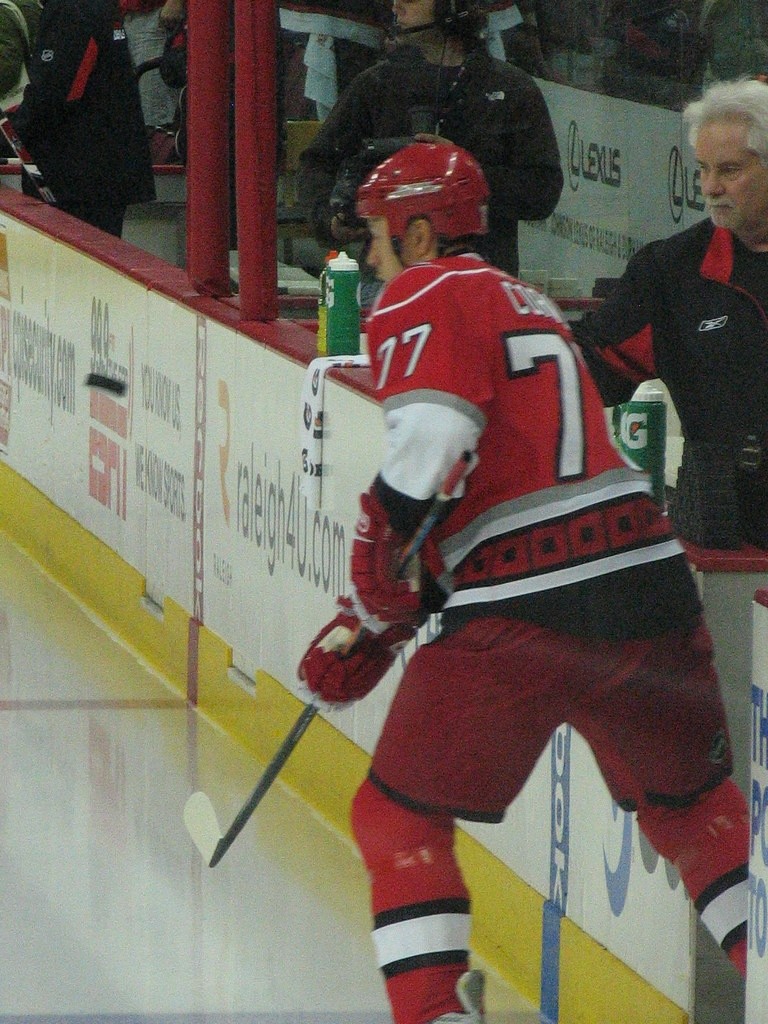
[350,475,436,625]
[296,557,448,704]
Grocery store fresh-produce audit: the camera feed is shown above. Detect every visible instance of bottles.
[613,382,670,518]
[316,251,359,358]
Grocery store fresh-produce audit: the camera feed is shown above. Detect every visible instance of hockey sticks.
[0,105,58,205]
[181,448,476,871]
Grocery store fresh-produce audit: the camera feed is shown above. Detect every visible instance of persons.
[0,0,767,552]
[297,143,752,1024]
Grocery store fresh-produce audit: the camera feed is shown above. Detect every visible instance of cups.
[286,120,322,172]
[519,269,581,320]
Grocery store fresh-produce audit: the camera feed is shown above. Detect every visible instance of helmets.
[354,144,489,242]
